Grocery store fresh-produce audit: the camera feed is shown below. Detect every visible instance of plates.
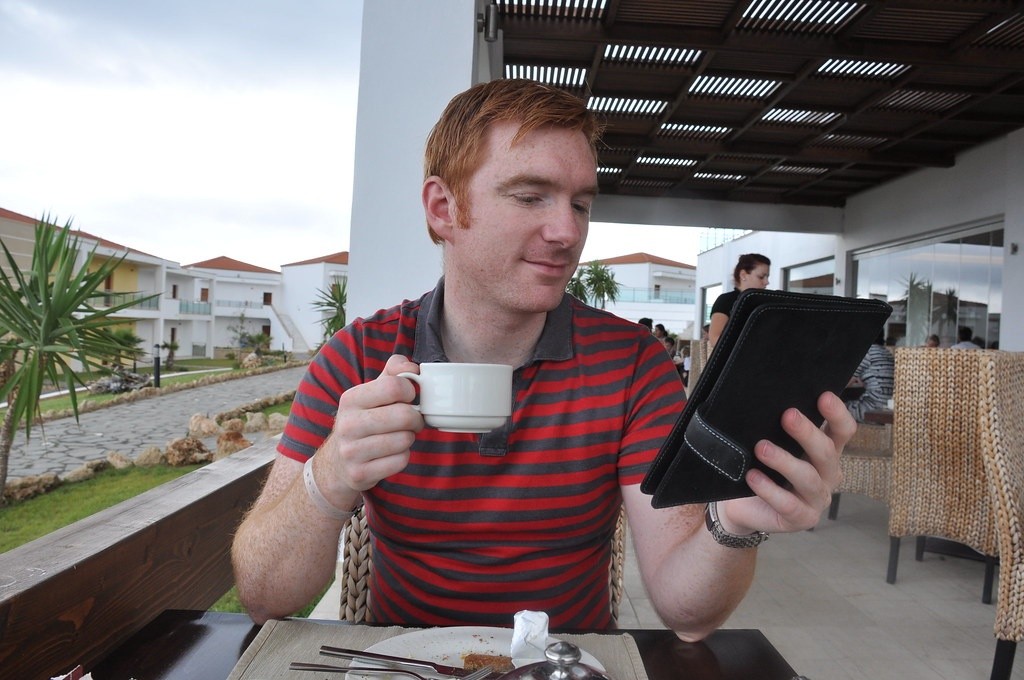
[345,626,607,680]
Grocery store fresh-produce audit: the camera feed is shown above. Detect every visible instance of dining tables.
[46,608,803,680]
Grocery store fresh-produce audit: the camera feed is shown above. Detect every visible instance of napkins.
[511,612,549,658]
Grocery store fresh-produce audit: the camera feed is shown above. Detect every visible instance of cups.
[399,363,513,433]
[497,642,614,680]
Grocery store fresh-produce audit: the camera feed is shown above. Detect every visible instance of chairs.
[337,504,627,628]
[827,420,893,519]
[886,347,998,604]
[978,348,1024,680]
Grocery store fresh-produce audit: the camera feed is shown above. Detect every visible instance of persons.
[231,79,857,642]
[709,254,771,347]
[639,317,709,387]
[845,328,999,419]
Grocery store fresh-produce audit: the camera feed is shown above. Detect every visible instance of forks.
[289,662,492,680]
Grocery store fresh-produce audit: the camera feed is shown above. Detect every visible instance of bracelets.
[303,456,358,521]
[704,502,769,549]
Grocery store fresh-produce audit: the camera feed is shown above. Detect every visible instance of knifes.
[320,646,506,680]
